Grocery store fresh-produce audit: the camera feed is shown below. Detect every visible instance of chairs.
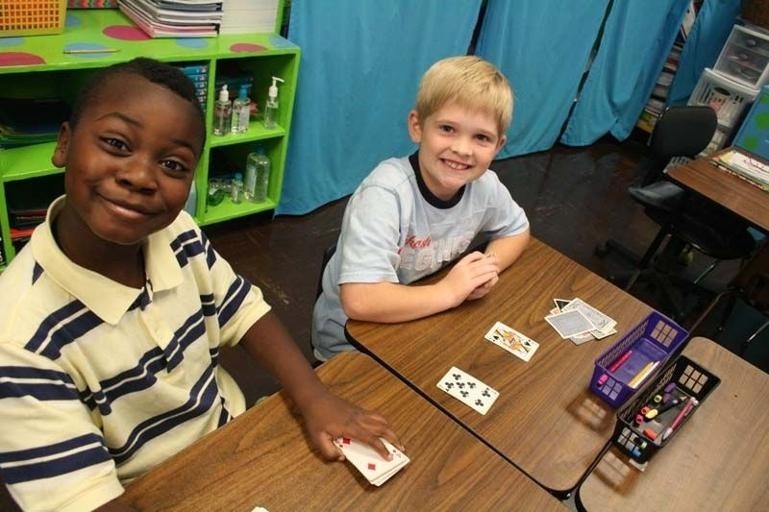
[601,105,757,326]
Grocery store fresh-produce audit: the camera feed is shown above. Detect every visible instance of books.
[637,4,699,137]
[115,0,224,40]
[5,190,67,242]
[708,147,769,195]
[0,96,75,147]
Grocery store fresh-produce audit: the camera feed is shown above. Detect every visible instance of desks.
[667,145,768,236]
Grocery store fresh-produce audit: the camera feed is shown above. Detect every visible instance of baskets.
[611,354,721,464]
[663,70,758,175]
[0,0,68,38]
[590,310,690,409]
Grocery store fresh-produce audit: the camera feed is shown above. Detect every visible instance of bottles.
[243,146,272,204]
[229,173,245,204]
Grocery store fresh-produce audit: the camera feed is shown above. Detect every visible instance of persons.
[0,54,408,509]
[310,53,532,372]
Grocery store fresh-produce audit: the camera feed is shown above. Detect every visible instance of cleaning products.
[212,85,232,136]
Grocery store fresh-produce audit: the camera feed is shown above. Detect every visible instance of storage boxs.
[686,20,769,161]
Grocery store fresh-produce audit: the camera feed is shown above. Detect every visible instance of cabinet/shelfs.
[1,9,301,266]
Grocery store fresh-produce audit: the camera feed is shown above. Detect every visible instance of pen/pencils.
[597,349,633,386]
[63,47,121,55]
[627,359,661,389]
[642,395,697,440]
